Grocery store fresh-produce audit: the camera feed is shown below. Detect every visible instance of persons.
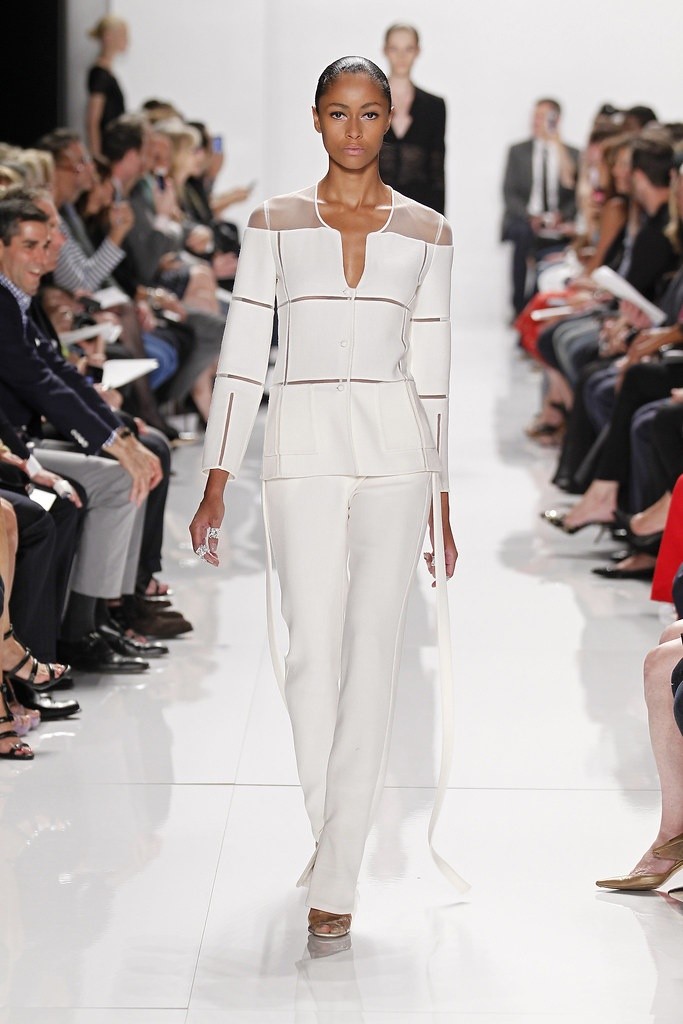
[378,23,446,216]
[0,15,253,761]
[186,56,458,959]
[498,99,683,890]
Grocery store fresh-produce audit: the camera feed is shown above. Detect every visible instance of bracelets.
[120,428,133,439]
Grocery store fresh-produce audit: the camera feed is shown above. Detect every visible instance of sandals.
[306,908,355,941]
[133,573,163,597]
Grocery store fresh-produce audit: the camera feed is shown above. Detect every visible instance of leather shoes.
[61,592,188,678]
[591,510,663,587]
[26,688,79,726]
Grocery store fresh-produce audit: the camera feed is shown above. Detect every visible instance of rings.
[196,543,209,558]
[209,528,219,540]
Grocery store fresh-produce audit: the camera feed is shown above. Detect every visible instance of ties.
[542,150,549,211]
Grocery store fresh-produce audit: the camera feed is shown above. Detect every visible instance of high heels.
[594,827,683,894]
[1,624,80,761]
[539,504,620,547]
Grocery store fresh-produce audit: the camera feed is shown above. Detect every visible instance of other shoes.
[525,398,568,436]
